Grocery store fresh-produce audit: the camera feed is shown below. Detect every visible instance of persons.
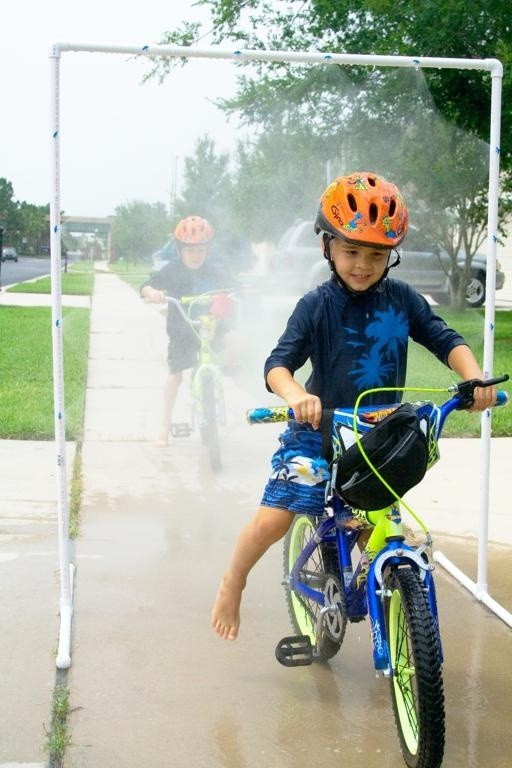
[139,214,265,472]
[207,172,496,640]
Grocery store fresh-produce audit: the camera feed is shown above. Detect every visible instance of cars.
[1,247,18,262]
[268,218,505,308]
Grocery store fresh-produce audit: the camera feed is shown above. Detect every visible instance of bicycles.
[144,284,248,474]
[247,374,510,767]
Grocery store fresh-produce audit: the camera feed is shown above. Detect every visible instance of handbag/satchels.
[328,400,430,510]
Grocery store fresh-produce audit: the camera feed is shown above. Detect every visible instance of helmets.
[173,216,213,245]
[313,170,408,248]
